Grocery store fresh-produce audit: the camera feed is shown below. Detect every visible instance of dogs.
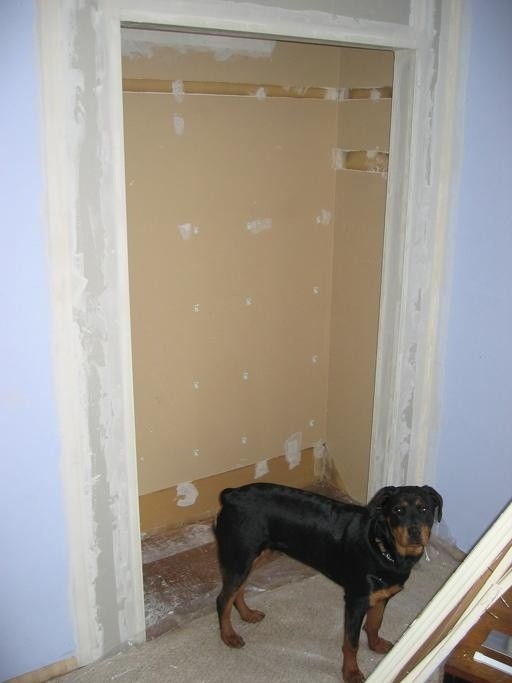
[211,482,443,683]
